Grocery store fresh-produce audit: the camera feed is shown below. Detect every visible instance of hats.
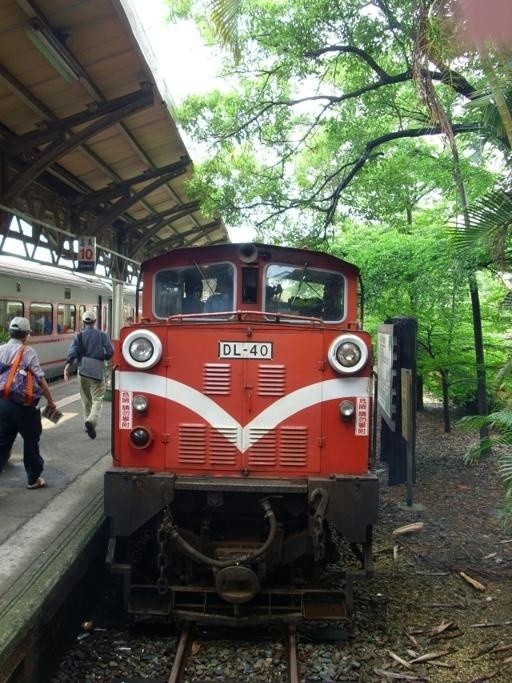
[82,310,96,322]
[9,316,33,333]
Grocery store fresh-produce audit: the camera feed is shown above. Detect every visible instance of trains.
[102,243,380,634]
[0,261,143,380]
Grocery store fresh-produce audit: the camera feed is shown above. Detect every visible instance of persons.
[62,309,115,439]
[180,277,206,314]
[0,314,56,488]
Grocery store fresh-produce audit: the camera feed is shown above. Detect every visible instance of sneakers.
[85,419,96,438]
[27,476,48,488]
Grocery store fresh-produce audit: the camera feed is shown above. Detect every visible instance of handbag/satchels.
[78,355,104,380]
[0,366,42,408]
